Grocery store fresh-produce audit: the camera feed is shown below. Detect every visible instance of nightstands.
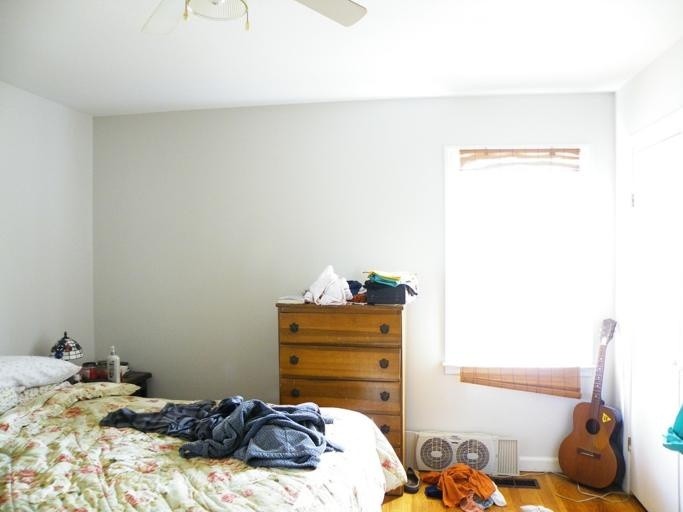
[76,357,152,398]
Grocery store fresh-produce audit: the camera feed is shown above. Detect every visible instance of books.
[275,296,305,305]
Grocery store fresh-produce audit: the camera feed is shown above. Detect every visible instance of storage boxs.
[361,279,405,304]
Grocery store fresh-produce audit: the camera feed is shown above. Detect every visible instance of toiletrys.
[79,345,128,382]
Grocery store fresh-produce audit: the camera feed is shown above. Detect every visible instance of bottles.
[120,362,128,376]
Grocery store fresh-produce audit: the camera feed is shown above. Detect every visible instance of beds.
[0,375,392,511]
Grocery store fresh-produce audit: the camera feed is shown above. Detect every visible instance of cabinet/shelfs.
[275,297,406,467]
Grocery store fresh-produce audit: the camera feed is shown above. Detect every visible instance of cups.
[89,366,96,379]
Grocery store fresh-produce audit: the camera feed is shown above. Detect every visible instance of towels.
[302,262,354,305]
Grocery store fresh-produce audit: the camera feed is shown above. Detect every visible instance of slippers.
[404,466,421,493]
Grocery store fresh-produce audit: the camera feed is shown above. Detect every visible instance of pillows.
[0,354,83,388]
[0,380,19,416]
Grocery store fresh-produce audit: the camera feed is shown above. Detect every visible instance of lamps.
[50,330,82,373]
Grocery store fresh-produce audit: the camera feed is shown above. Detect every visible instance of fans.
[177,0,368,32]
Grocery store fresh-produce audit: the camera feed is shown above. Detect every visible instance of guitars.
[558,319,624,490]
